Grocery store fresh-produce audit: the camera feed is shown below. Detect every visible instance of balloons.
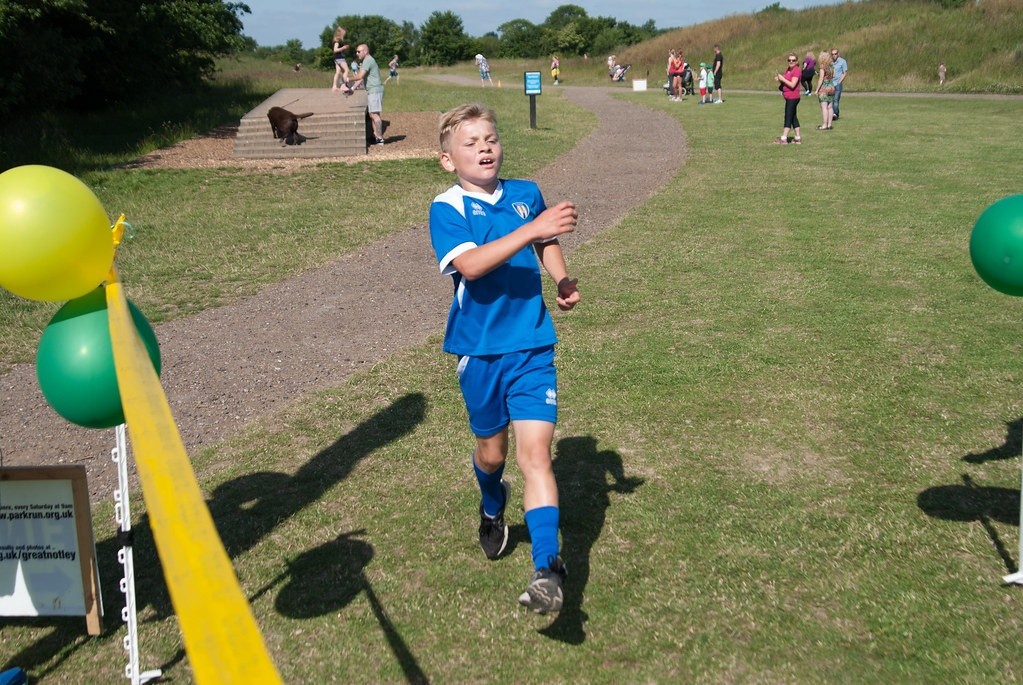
[971,194,1023,297]
[33,282,163,429]
[0,163,115,304]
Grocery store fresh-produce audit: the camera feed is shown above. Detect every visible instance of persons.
[475,54,494,86]
[830,48,849,119]
[607,55,625,81]
[351,60,359,75]
[706,65,715,103]
[712,44,725,103]
[695,63,706,103]
[937,62,947,86]
[428,103,583,608]
[551,55,560,85]
[340,44,387,145]
[800,51,816,96]
[664,47,686,101]
[773,51,803,142]
[383,55,400,85]
[814,49,835,130]
[331,26,351,91]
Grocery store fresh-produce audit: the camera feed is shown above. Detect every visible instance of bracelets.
[347,78,349,83]
[350,87,355,91]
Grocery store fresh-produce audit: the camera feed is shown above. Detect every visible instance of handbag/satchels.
[779,82,784,91]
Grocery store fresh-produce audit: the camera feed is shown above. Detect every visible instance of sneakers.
[519,568,563,632]
[479,480,511,561]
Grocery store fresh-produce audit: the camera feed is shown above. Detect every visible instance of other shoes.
[833,114,838,121]
[790,138,801,145]
[773,138,788,144]
[816,125,828,130]
[699,100,705,103]
[805,90,809,94]
[675,98,682,101]
[368,138,384,146]
[808,93,812,96]
[714,100,723,104]
[706,99,713,103]
[341,86,350,92]
[553,82,559,85]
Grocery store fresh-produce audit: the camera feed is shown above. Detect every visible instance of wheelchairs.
[664,68,695,96]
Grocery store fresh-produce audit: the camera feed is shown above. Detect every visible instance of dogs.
[267,106,314,147]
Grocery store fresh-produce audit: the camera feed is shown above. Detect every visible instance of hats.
[699,63,706,67]
[707,65,713,68]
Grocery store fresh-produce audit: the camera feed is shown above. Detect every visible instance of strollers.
[610,64,631,82]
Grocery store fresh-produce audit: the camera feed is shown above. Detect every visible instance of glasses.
[787,59,797,62]
[832,53,838,55]
[356,51,360,53]
